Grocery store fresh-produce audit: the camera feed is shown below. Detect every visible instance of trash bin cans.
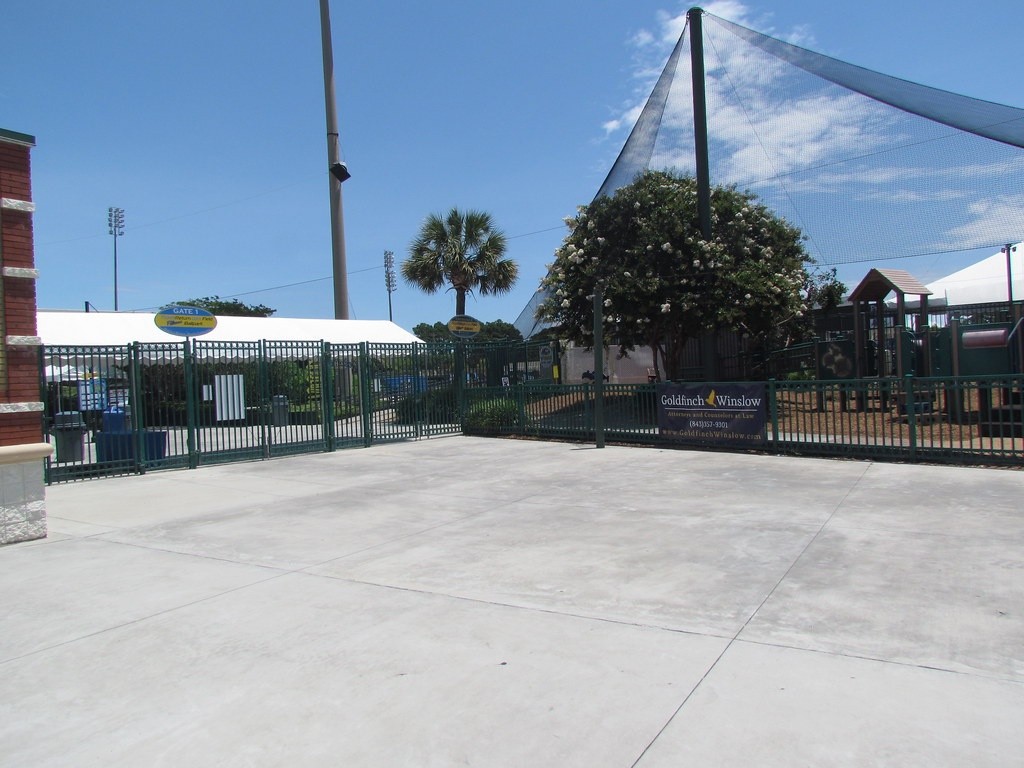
[119,405,133,432]
[273,395,289,426]
[54,411,87,464]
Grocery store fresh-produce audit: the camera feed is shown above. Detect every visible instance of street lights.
[1001,241,1018,368]
[107,206,125,309]
[383,249,398,321]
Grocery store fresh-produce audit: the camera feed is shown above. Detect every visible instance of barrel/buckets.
[92,429,167,462]
